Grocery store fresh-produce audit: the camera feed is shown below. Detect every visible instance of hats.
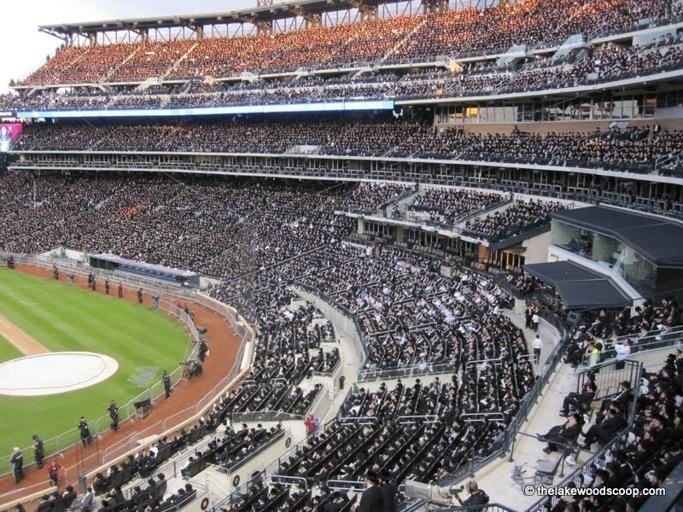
[11,342,683,512]
